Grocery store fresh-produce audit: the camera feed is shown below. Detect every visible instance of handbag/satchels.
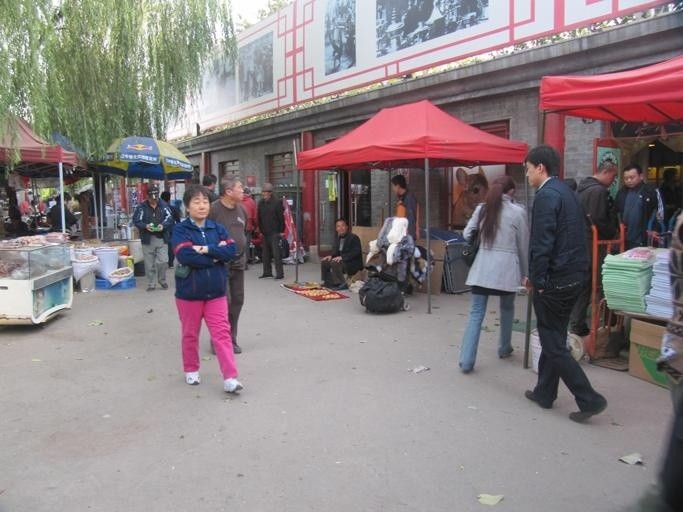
[278,238,289,258]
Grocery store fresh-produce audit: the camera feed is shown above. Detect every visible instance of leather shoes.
[333,282,348,290]
[526,389,553,410]
[569,397,608,422]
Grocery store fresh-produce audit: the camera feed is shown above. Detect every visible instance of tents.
[538,55,683,180]
[298,99,530,312]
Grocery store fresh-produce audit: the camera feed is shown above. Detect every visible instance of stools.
[344,268,365,288]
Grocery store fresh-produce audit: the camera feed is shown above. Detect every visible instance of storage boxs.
[628,318,674,390]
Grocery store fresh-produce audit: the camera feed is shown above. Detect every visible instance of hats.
[148,187,159,199]
[261,183,274,192]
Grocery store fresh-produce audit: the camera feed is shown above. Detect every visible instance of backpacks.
[358,276,403,314]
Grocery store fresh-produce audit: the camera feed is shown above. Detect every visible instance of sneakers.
[168,261,174,268]
[223,378,243,393]
[158,280,168,290]
[231,341,241,355]
[147,283,156,290]
[258,272,285,280]
[185,370,201,387]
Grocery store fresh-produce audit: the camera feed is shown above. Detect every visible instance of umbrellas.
[97,137,195,182]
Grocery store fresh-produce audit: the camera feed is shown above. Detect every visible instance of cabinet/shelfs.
[0,242,73,325]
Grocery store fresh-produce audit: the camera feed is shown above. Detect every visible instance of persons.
[132,174,286,392]
[458,142,683,510]
[390,175,420,284]
[321,217,363,290]
[47,192,78,236]
